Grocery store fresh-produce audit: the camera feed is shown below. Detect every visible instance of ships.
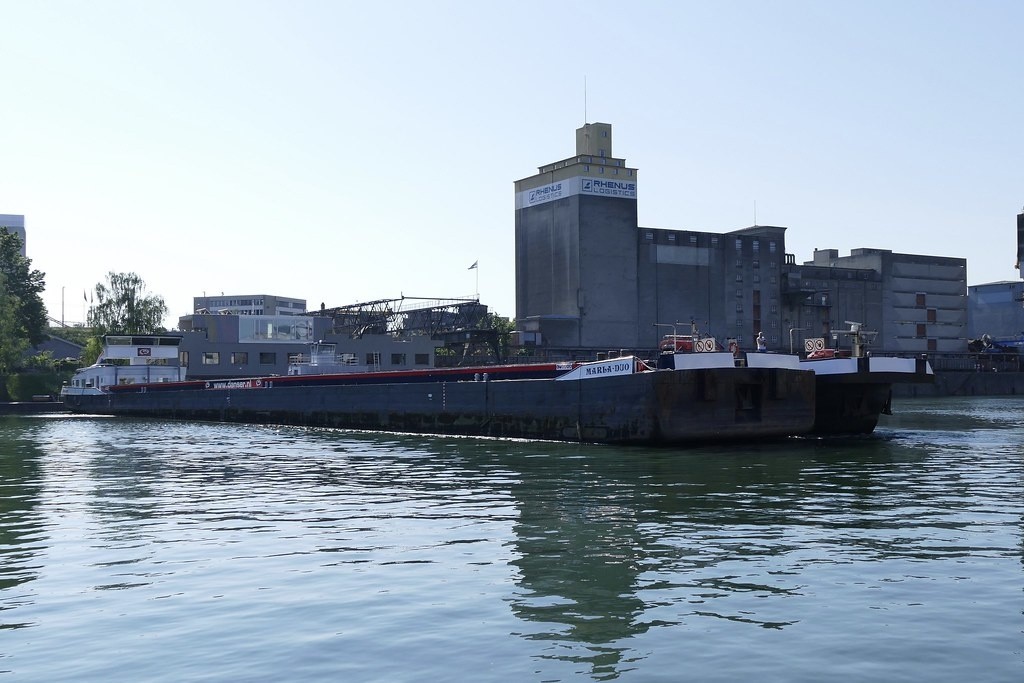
[59,296,936,443]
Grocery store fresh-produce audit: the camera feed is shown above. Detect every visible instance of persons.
[757,332,767,352]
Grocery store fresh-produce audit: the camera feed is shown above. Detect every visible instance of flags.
[468,261,477,270]
